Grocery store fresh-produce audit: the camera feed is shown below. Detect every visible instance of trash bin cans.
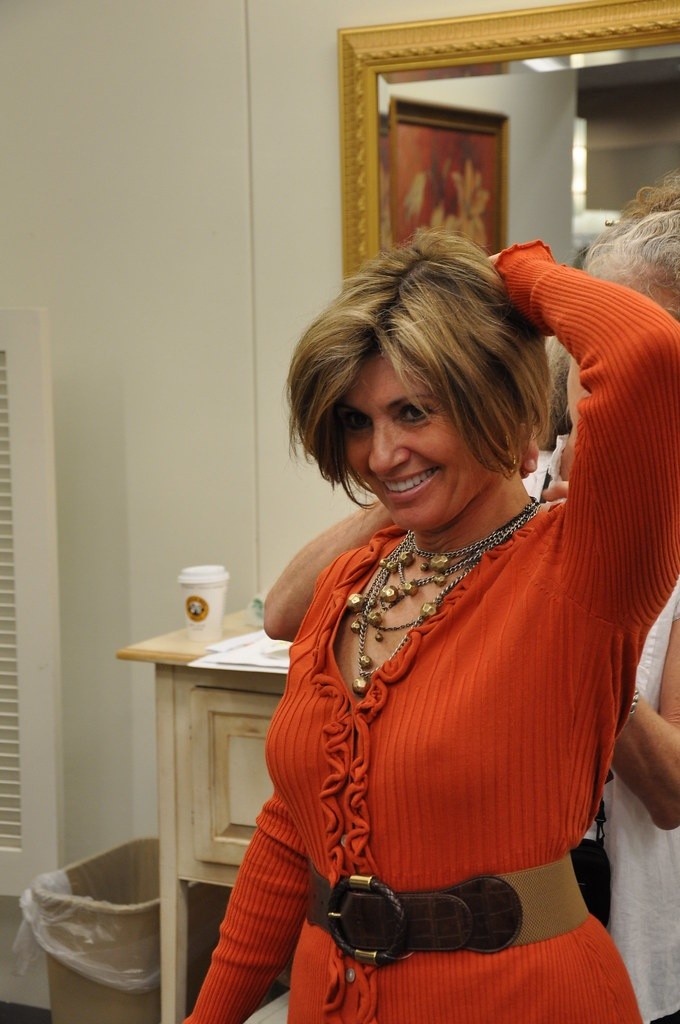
[33,836,229,1024]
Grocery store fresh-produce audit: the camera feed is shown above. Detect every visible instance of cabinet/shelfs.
[116,609,289,1024]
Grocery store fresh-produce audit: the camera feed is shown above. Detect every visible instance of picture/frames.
[387,96,509,260]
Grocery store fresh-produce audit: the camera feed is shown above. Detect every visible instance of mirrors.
[337,0,680,373]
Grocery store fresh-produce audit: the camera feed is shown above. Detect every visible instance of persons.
[264,169,680,1024]
[178,227,680,1024]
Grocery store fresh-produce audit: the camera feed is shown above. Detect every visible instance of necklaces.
[345,496,539,692]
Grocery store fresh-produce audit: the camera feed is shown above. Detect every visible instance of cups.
[177,564,229,640]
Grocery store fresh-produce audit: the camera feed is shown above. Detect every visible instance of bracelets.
[627,688,639,727]
